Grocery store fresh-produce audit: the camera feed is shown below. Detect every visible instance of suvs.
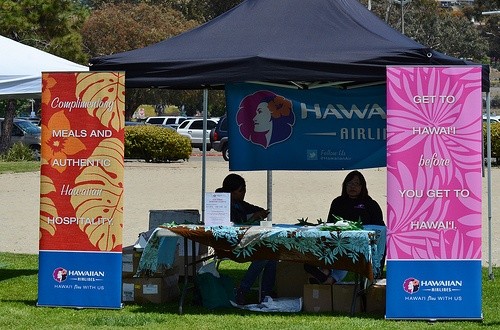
[210,116,229,161]
[175,119,220,151]
[143,116,188,130]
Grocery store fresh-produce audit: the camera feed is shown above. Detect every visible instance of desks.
[133,222,377,318]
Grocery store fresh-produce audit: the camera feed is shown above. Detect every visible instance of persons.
[216,173,280,305]
[308,170,386,285]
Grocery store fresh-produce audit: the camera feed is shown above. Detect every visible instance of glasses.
[347,181,362,186]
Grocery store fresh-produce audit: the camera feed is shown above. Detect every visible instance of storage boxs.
[275,258,387,312]
[123,245,182,301]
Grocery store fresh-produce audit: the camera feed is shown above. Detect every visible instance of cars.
[482,115,500,124]
[0,118,41,161]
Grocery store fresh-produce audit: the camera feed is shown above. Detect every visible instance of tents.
[89,0,491,280]
[0,34,89,94]
